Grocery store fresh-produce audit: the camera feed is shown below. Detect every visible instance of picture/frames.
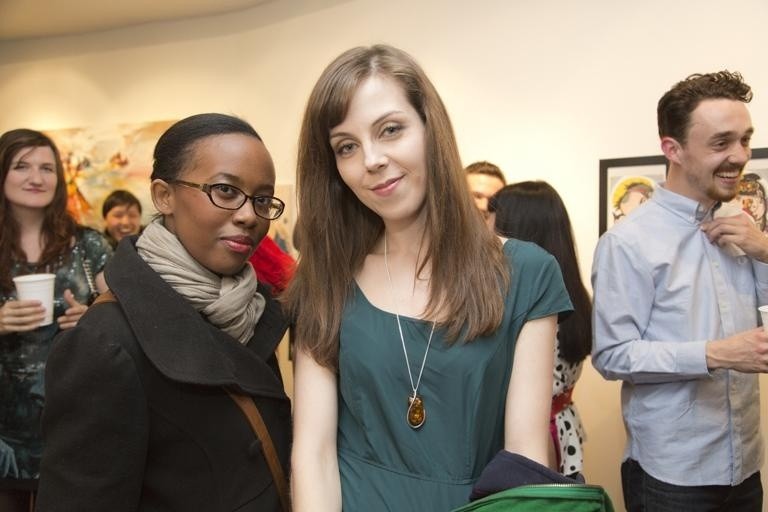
[598,147,768,244]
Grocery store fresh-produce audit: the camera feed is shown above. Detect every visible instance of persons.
[590,69,768,512]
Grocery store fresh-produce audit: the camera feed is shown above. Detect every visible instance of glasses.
[165,177,286,222]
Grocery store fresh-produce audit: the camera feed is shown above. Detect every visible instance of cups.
[758,304,768,338]
[12,272,56,328]
